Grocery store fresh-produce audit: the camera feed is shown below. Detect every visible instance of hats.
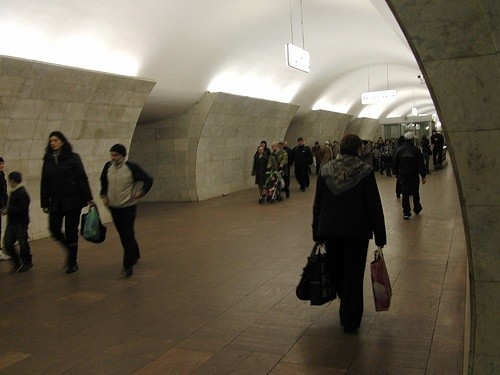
[432,129,436,133]
[315,141,319,144]
[110,143,127,157]
[404,131,418,140]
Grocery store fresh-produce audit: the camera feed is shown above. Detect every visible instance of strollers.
[258,167,285,204]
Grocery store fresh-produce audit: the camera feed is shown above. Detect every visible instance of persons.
[251,128,444,221]
[311,134,387,335]
[40,131,96,272]
[0,157,33,272]
[100,144,154,277]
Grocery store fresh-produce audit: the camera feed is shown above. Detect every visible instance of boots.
[0,248,11,260]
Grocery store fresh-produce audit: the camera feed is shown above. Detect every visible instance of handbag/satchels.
[80,204,107,244]
[296,243,337,306]
[370,246,392,312]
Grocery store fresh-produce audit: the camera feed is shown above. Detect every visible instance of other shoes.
[380,171,383,175]
[11,263,23,274]
[133,256,140,265]
[19,263,33,272]
[302,187,305,192]
[125,266,133,276]
[403,215,410,219]
[397,193,400,198]
[66,265,78,273]
[416,206,422,215]
[285,190,289,198]
[340,321,358,334]
